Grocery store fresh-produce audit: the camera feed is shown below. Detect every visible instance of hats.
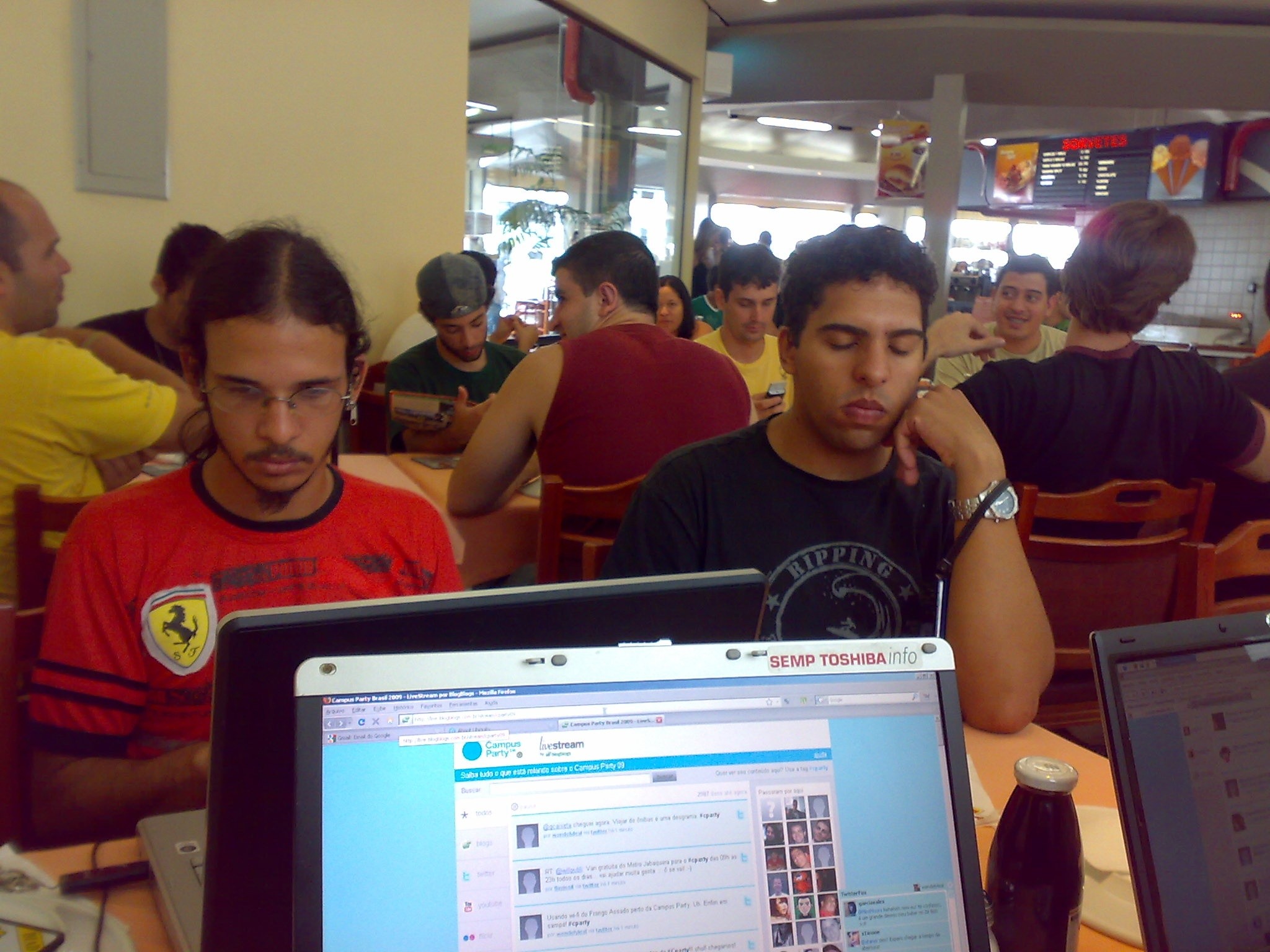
[417,253,487,317]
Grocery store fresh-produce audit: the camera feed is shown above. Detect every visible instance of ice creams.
[1151,134,1208,196]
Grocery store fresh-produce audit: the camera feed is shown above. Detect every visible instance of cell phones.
[766,381,787,400]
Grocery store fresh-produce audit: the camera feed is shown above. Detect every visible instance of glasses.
[200,372,353,418]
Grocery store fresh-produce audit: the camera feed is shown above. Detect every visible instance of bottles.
[984,897,999,952]
[986,756,1084,952]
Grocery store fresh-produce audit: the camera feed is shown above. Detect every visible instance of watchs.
[948,479,1019,523]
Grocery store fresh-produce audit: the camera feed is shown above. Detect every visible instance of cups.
[548,286,559,321]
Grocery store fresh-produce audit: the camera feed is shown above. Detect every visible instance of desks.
[24,711,1153,952]
[113,450,541,592]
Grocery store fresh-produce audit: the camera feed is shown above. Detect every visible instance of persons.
[921,309,1006,376]
[931,254,1069,390]
[1040,268,1070,330]
[919,200,1270,540]
[383,252,535,455]
[1209,267,1269,599]
[385,247,540,364]
[0,178,210,602]
[445,233,749,585]
[29,227,466,849]
[692,244,799,422]
[71,219,229,375]
[655,217,786,343]
[575,225,1056,728]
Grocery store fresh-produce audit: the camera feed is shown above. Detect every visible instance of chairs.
[0,482,100,855]
[511,300,550,336]
[1011,474,1270,673]
[349,360,398,455]
[532,473,651,587]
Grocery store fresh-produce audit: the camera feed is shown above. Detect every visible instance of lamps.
[463,209,492,252]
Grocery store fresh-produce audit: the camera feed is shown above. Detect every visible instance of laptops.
[1089,608,1270,952]
[138,570,989,952]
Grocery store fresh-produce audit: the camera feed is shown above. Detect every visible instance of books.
[389,389,477,433]
